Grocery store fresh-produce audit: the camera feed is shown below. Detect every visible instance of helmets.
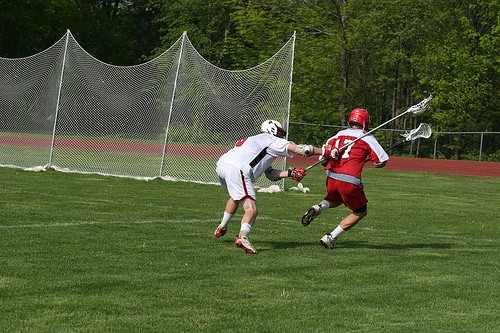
[348,108,371,132]
[261,120,287,137]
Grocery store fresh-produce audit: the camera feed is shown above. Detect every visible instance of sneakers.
[234,234,258,254]
[213,224,227,238]
[302,204,322,226]
[320,233,336,250]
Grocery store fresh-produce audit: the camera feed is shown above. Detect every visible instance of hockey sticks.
[305,95,433,172]
[364,122,431,163]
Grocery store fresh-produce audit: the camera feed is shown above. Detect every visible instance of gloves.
[321,144,342,161]
[288,166,308,182]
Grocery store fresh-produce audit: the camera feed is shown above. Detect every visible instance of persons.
[301,108,389,249]
[213,120,339,254]
